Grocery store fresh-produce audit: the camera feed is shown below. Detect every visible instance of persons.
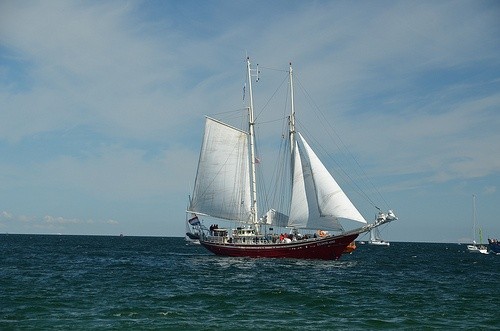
[273,232,320,243]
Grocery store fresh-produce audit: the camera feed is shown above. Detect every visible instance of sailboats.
[187,57,398,258]
[358,214,391,247]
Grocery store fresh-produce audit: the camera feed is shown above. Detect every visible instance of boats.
[467,194,500,256]
